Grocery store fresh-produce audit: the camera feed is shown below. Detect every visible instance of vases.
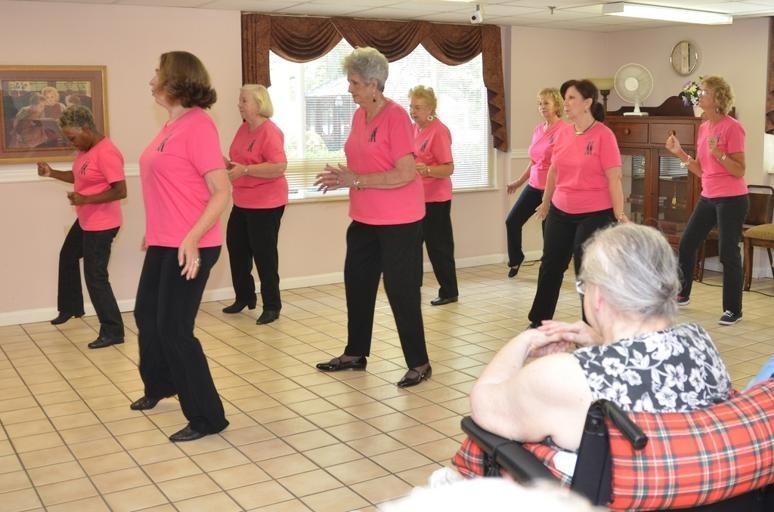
[691,105,706,118]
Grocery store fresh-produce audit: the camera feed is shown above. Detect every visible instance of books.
[631,194,668,207]
[658,219,687,257]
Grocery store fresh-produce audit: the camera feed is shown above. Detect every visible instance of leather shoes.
[88,334,124,348]
[397,367,432,387]
[51,309,85,324]
[526,321,542,330]
[169,423,206,441]
[317,355,367,371]
[131,391,176,410]
[431,296,458,305]
[508,255,524,277]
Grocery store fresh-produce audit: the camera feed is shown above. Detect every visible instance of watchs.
[716,151,727,163]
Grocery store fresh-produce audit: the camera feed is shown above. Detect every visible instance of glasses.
[576,281,585,294]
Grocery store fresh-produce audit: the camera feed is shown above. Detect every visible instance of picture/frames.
[0,63,113,169]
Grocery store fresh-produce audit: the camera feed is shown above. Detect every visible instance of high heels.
[256,311,279,325]
[223,293,256,313]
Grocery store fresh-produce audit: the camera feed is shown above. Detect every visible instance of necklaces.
[574,119,597,136]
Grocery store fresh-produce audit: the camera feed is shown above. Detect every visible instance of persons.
[220,82,291,325]
[405,83,460,305]
[526,77,630,329]
[40,86,66,148]
[9,92,49,148]
[312,45,434,390]
[35,102,128,350]
[126,50,234,445]
[65,94,82,107]
[450,220,733,489]
[504,87,571,279]
[664,74,752,327]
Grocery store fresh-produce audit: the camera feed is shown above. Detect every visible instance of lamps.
[601,2,735,28]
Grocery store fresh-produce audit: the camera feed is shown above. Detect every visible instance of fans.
[611,61,656,117]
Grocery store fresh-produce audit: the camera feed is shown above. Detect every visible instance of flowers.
[678,79,699,108]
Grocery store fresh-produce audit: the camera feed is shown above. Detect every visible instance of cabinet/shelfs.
[602,95,737,284]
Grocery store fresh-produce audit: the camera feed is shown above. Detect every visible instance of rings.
[336,178,340,186]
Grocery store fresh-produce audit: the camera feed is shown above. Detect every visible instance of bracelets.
[679,154,692,169]
[426,166,432,177]
[352,174,363,191]
[244,164,249,176]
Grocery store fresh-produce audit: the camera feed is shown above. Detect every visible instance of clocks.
[670,39,700,75]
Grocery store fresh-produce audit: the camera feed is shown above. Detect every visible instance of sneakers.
[718,310,742,324]
[678,296,690,306]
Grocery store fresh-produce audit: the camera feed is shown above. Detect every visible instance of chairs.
[697,184,774,284]
[741,223,774,292]
